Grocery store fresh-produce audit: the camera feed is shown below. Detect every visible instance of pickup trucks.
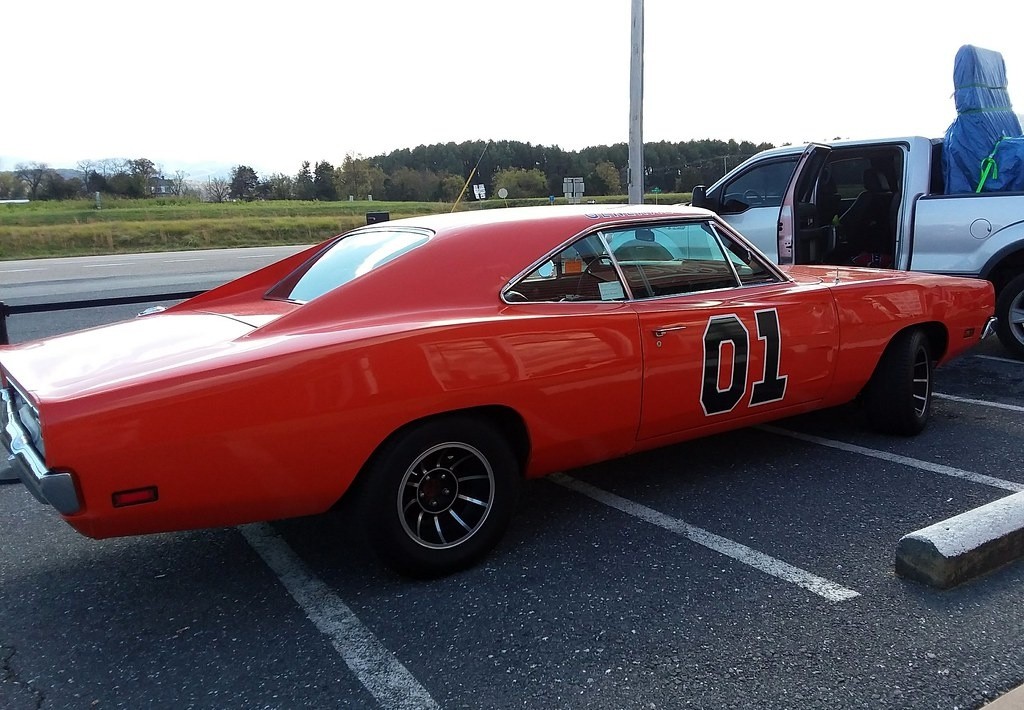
[600,135,1024,364]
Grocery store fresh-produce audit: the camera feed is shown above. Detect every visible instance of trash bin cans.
[366,213,390,224]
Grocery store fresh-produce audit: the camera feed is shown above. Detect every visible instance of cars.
[0,203,1000,583]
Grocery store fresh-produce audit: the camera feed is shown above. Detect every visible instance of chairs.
[831,162,895,267]
